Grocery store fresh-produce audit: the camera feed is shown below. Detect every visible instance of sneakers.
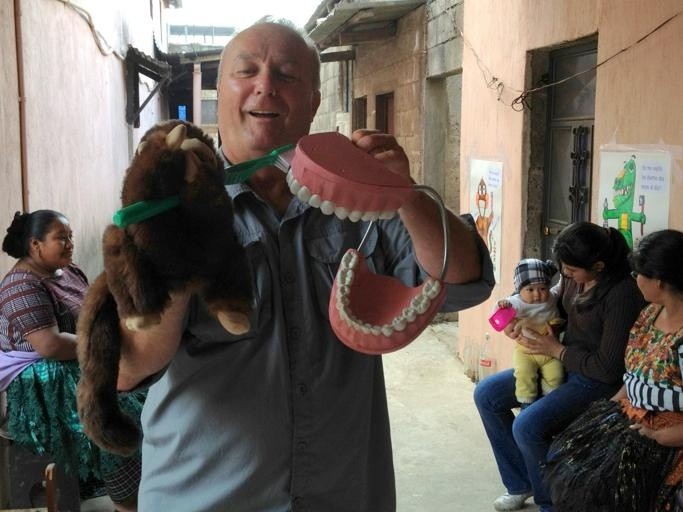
[492,491,534,511]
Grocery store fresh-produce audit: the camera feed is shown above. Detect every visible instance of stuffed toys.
[76,119,253,456]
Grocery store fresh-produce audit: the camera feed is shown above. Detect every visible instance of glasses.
[630,270,639,279]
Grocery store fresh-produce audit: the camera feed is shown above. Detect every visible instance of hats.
[512,258,558,292]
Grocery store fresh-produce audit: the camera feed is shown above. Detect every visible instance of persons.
[0,208,144,511]
[541,228,682,511]
[96,15,497,511]
[471,221,654,512]
[487,258,563,408]
